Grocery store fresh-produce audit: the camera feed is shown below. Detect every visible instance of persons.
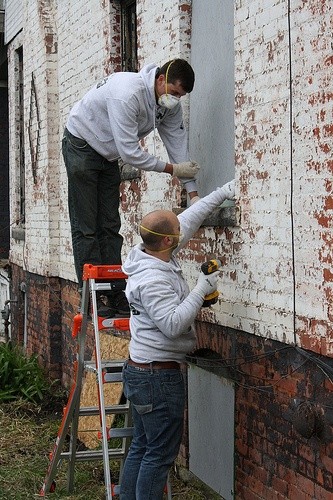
[119,179,236,500]
[61,58,199,318]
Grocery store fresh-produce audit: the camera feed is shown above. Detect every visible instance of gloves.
[189,196,199,204]
[221,179,235,200]
[172,160,200,178]
[192,271,220,297]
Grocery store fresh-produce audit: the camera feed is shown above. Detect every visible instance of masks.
[158,92,180,109]
[172,231,184,247]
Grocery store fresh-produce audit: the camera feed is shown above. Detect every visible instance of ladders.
[38,265,172,500]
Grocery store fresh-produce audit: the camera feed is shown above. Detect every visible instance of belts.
[129,358,180,371]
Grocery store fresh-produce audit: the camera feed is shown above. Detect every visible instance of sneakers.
[105,296,131,315]
[77,295,115,317]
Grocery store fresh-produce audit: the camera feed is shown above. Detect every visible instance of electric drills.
[201,257,226,308]
